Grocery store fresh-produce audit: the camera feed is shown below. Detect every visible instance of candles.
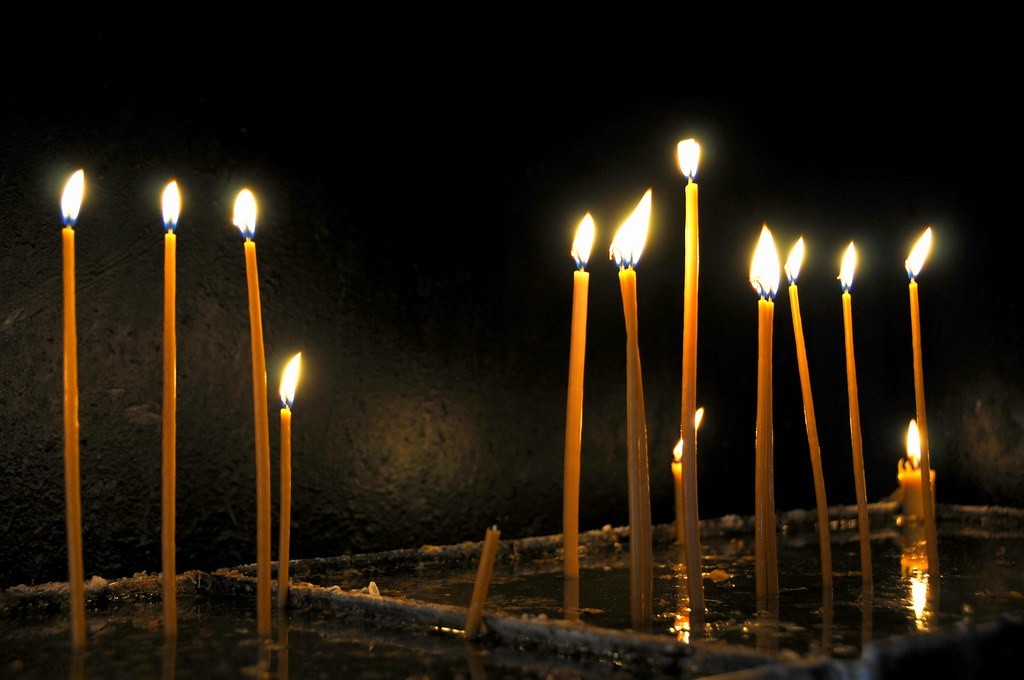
[231,187,272,637]
[819,588,836,657]
[750,221,783,599]
[902,226,940,581]
[859,582,875,657]
[894,420,938,521]
[769,600,780,660]
[690,613,707,639]
[781,236,835,589]
[467,525,500,638]
[60,167,89,652]
[645,623,653,636]
[671,134,704,613]
[754,602,770,658]
[159,179,183,634]
[277,351,304,611]
[632,624,644,635]
[838,241,872,582]
[561,189,656,623]
